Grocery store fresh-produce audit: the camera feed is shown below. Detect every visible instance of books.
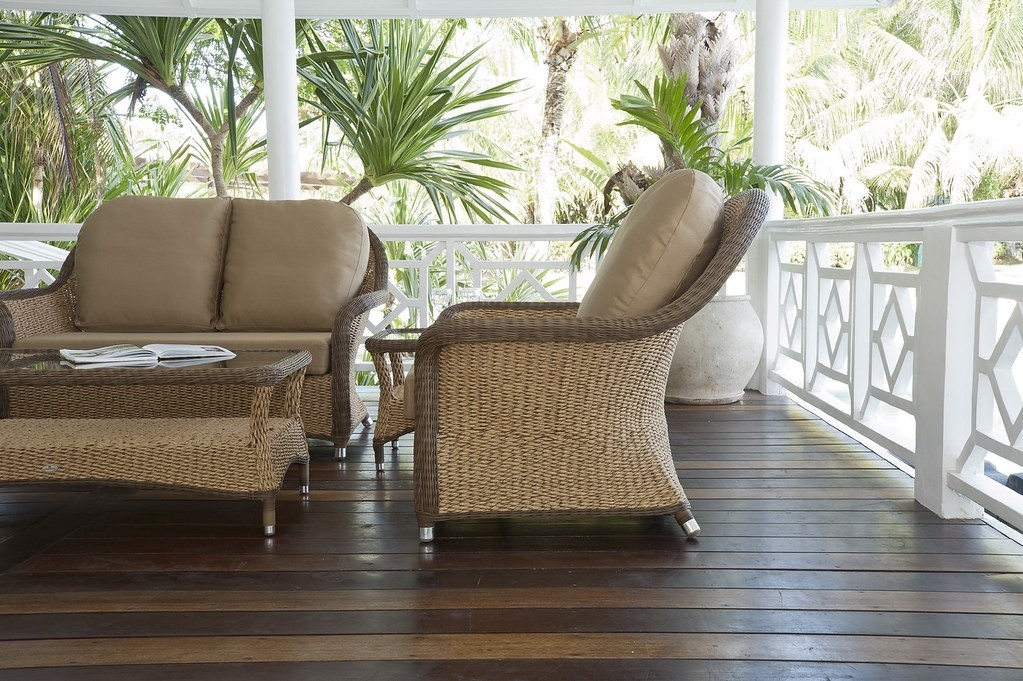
[58,343,237,364]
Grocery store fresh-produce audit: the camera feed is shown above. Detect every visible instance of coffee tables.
[1,344,313,534]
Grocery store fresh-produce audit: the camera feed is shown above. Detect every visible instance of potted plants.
[566,71,838,403]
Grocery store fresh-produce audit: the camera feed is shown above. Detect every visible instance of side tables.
[365,327,433,471]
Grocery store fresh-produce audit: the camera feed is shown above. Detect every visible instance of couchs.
[0,195,392,464]
[409,165,772,544]
